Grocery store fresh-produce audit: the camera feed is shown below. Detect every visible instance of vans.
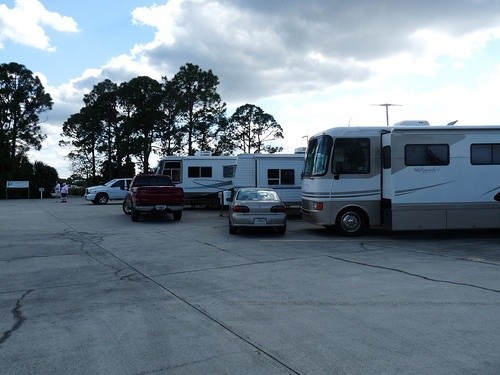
[155,152,237,211]
[225,147,306,221]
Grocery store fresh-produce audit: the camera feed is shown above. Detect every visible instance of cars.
[226,188,287,236]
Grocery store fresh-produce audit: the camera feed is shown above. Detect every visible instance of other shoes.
[60,201,66,203]
[56,201,58,202]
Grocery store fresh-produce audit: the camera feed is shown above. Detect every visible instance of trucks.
[299,120,500,238]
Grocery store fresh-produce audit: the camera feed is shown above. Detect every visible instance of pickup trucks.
[123,173,184,222]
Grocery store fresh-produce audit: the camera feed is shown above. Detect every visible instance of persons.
[53,180,69,203]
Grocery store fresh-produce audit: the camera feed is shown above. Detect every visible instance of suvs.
[84,178,133,205]
